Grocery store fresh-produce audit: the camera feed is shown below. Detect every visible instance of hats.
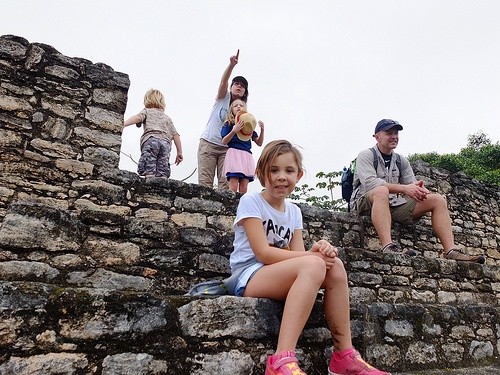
[235,110,257,141]
[372,119,403,137]
[232,76,248,86]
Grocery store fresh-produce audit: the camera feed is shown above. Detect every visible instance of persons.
[349,120,486,264]
[197,49,249,193]
[220,99,264,192]
[122,88,183,179]
[229,140,392,375]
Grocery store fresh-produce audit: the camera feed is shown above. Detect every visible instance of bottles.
[342,166,348,182]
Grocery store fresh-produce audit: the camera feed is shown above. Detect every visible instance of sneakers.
[443,248,485,264]
[381,243,416,257]
[328,347,392,375]
[265,351,308,375]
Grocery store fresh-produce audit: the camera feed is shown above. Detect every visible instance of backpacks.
[342,147,400,212]
